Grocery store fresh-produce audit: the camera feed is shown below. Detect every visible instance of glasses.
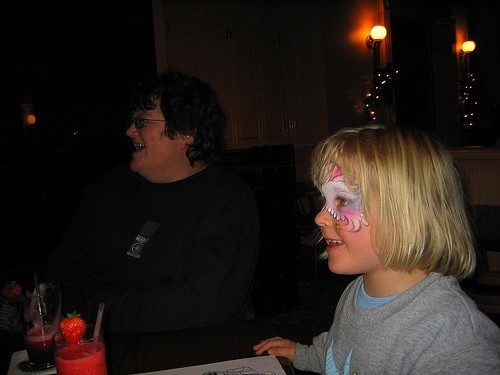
[127,115,166,129]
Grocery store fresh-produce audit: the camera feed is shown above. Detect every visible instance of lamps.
[366,25,387,70]
[461,40,478,81]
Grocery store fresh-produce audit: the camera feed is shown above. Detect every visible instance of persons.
[48,70,260,334]
[253,127,500,375]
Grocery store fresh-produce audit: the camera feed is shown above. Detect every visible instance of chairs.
[222,145,304,321]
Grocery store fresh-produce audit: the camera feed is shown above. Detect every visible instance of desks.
[0,319,298,375]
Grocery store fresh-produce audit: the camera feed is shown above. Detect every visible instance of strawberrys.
[3,279,21,300]
[60,311,87,345]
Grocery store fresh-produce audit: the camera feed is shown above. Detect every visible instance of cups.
[19,283,62,369]
[52,324,107,375]
